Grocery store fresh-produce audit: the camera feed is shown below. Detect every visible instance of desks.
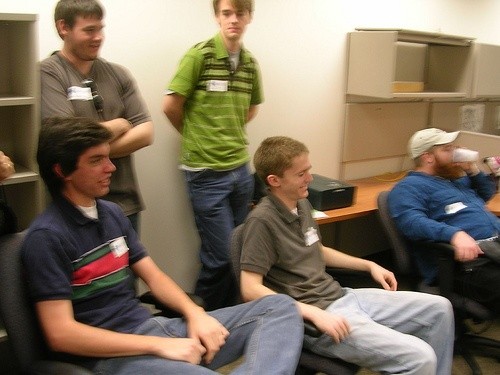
[313,169,500,224]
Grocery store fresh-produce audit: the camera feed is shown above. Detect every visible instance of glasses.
[84,76,105,113]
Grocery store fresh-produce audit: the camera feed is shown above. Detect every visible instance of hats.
[407,128,461,160]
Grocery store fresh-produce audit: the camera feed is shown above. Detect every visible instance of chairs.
[0,230,205,375]
[377,190,500,375]
[230,223,374,375]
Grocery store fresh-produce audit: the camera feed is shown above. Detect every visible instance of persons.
[383,128,500,317]
[162,0,265,310]
[39,0,152,304]
[17,117,303,375]
[241,137,454,375]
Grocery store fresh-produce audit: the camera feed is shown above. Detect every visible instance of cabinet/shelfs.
[0,12,45,237]
[347,31,500,103]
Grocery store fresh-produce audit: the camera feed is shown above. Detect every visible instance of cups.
[452,148,480,162]
